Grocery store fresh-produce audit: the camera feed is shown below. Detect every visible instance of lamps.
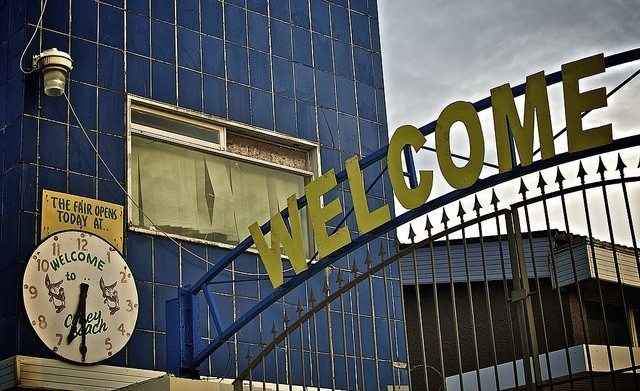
[31,47,74,98]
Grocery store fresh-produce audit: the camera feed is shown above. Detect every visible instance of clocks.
[22,229,140,366]
